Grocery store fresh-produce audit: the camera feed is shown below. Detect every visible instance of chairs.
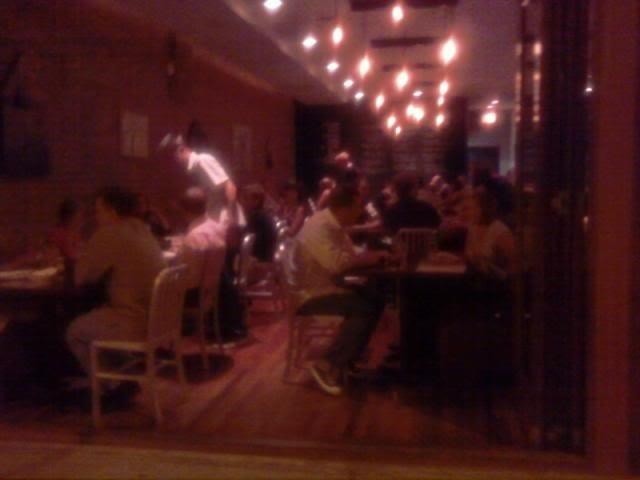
[273,219,348,386]
[396,228,437,267]
[87,261,190,432]
[231,234,257,297]
[177,242,228,375]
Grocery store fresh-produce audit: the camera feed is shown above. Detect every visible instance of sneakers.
[310,362,342,396]
